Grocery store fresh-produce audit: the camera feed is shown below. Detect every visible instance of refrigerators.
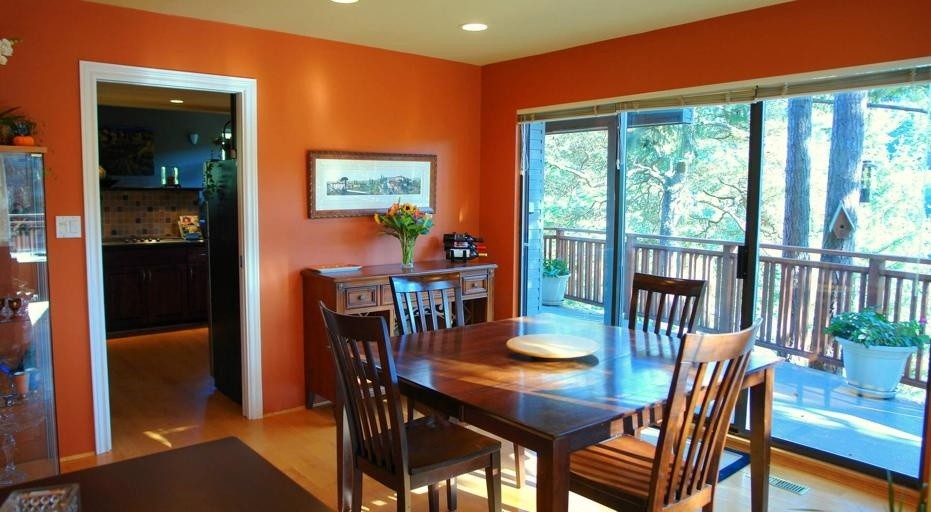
[204,158,242,406]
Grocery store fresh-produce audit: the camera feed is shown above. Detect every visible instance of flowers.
[368,196,438,265]
[185,126,202,135]
[825,310,930,351]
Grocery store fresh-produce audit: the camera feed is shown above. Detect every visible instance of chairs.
[316,296,504,512]
[629,269,712,341]
[566,315,765,512]
[387,271,527,493]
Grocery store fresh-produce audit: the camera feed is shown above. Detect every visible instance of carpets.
[639,422,753,485]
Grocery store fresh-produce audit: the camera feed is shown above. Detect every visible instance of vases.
[834,334,914,399]
[190,133,199,144]
[399,234,418,270]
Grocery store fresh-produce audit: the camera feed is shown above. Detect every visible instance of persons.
[182,217,199,237]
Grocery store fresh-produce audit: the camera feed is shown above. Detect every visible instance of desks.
[0,430,336,512]
[353,303,787,512]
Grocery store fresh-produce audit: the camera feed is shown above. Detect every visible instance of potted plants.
[539,255,574,310]
[213,119,236,160]
[9,119,35,145]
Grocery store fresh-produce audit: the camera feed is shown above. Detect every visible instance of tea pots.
[218,120,232,160]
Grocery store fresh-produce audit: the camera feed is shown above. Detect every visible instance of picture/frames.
[305,149,440,220]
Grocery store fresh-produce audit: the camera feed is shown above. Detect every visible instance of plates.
[503,332,600,362]
[308,262,363,274]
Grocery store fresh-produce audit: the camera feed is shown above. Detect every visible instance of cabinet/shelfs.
[105,242,192,338]
[191,237,211,335]
[0,136,63,484]
[297,256,499,421]
[200,195,243,403]
